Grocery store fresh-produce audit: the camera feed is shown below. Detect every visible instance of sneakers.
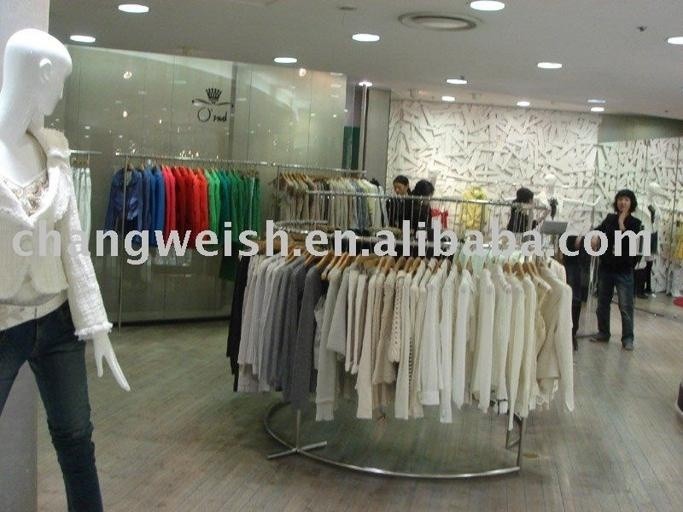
[675,383,683,414]
[622,336,633,350]
[572,336,579,351]
[638,293,647,299]
[590,330,612,342]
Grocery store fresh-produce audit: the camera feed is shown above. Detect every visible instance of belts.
[0,294,69,319]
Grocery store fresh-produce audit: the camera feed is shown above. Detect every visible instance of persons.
[588,189,645,351]
[506,187,550,234]
[382,175,412,229]
[563,233,599,351]
[638,182,662,299]
[411,180,435,229]
[0,28,130,512]
